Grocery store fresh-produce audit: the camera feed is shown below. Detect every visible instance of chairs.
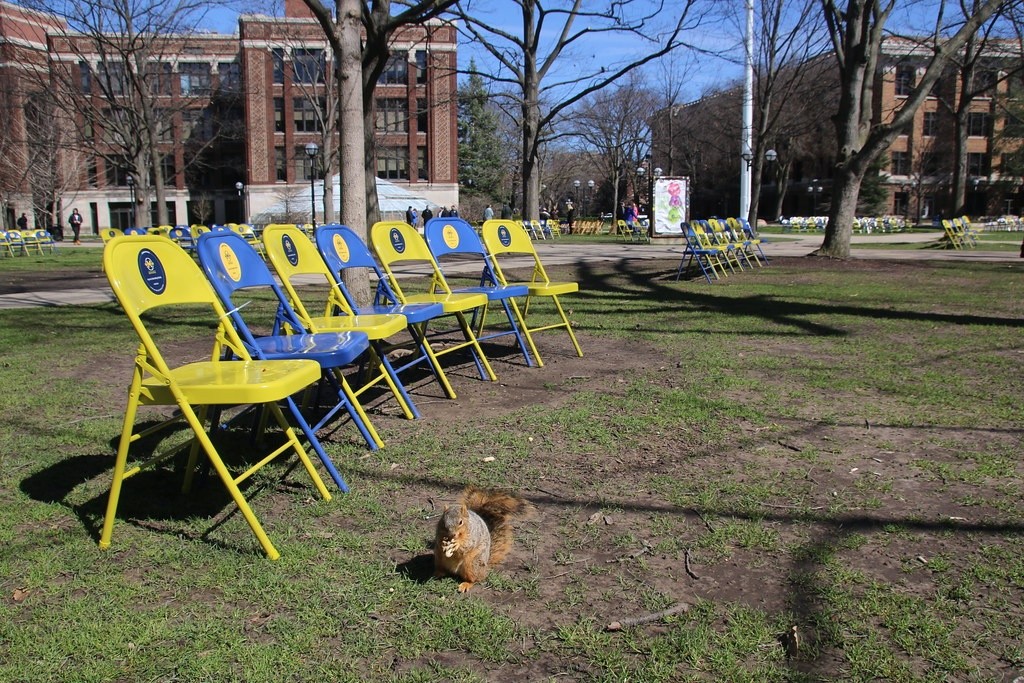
[0,229,59,258]
[98,219,652,562]
[779,215,1024,250]
[676,217,770,284]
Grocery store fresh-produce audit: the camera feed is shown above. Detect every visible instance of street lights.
[126,176,135,228]
[305,142,318,236]
[742,148,778,231]
[899,181,916,220]
[635,167,663,237]
[807,179,823,215]
[236,182,243,223]
[574,179,595,220]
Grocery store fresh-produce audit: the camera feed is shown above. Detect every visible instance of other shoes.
[73,239,81,245]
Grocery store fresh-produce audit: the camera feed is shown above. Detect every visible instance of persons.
[17,213,27,244]
[68,208,83,245]
[540,205,559,220]
[501,202,512,221]
[567,204,578,234]
[406,205,459,229]
[616,201,640,236]
[483,204,494,220]
[598,212,604,229]
